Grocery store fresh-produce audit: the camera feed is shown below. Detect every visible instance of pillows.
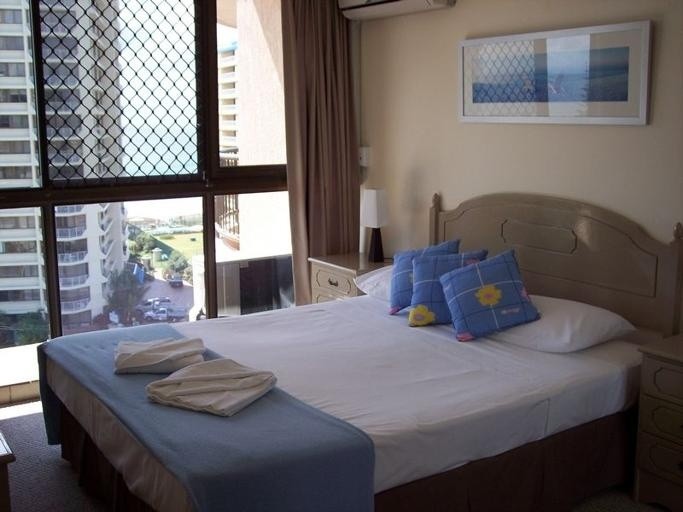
[353,238,637,354]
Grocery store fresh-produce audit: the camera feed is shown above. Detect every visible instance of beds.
[44,192,681,512]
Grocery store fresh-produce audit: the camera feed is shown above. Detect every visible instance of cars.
[168,274,183,287]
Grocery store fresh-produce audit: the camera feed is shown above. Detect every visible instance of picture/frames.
[459,19,652,125]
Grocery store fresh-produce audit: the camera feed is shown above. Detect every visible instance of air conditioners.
[337,1,453,22]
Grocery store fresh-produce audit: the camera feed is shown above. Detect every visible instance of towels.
[113,337,278,417]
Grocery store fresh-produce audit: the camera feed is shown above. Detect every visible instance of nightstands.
[306,252,393,302]
[634,331,682,510]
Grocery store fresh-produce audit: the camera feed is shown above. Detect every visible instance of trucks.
[133,296,186,322]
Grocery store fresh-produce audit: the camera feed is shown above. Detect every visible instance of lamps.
[360,188,388,262]
